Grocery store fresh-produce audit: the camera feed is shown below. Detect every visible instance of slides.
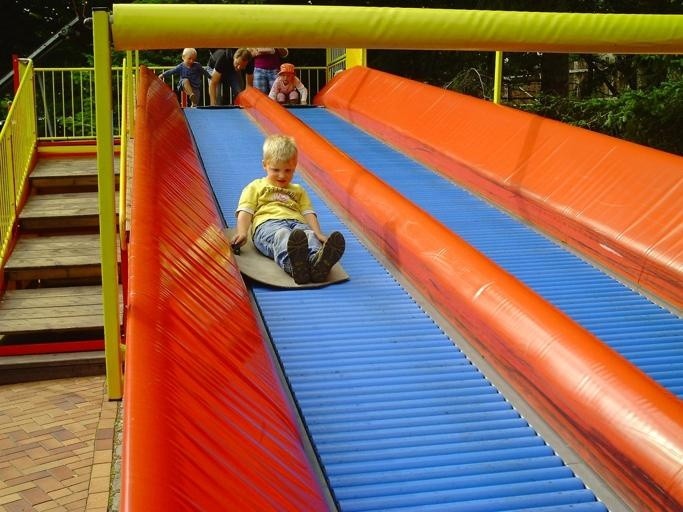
[128,64,683,511]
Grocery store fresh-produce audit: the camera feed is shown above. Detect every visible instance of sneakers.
[309,230,346,283]
[287,229,311,285]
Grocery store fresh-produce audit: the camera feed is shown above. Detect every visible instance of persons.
[267,63,306,106]
[206,48,255,106]
[157,47,213,108]
[246,47,289,95]
[228,133,345,285]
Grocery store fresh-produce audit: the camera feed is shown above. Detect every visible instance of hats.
[278,63,296,75]
